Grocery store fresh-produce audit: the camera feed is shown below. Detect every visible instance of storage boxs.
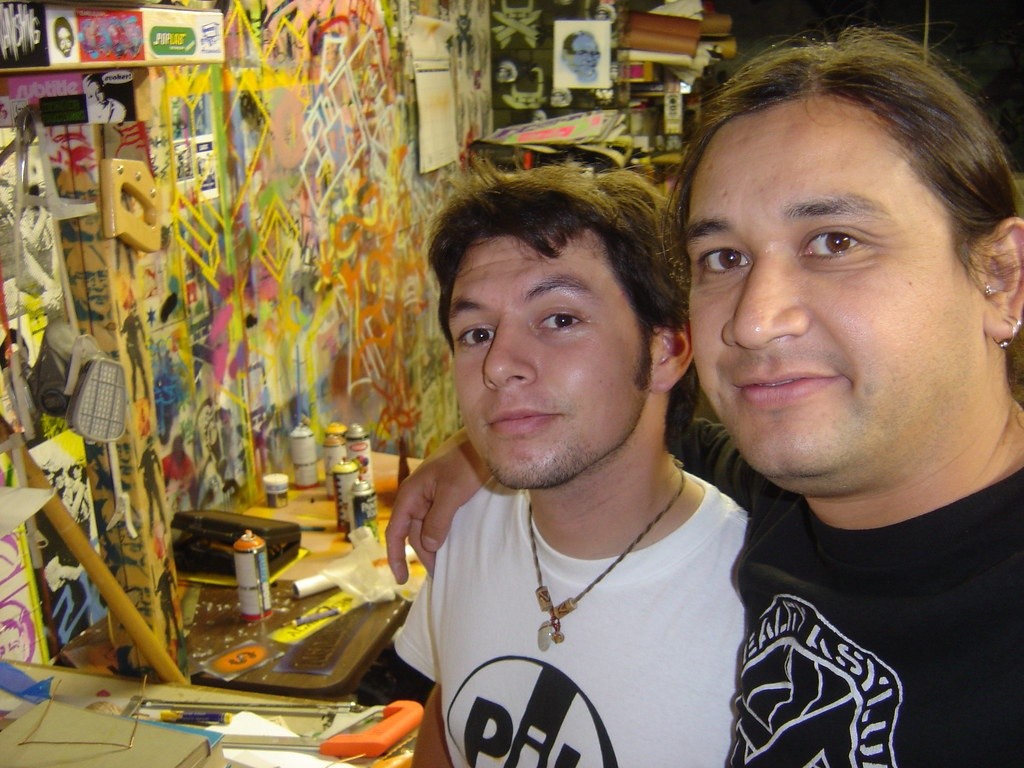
[171,510,302,580]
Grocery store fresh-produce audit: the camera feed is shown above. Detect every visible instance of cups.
[262,473,289,509]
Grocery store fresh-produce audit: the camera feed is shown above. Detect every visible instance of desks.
[0,451,429,768]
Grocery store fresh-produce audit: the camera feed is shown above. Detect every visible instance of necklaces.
[528,470,685,651]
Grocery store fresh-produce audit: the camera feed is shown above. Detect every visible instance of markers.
[161,710,233,725]
[292,609,339,627]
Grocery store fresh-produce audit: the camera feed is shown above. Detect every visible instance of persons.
[95,429,155,629]
[385,29,1024,768]
[66,245,114,322]
[83,74,127,123]
[393,161,751,768]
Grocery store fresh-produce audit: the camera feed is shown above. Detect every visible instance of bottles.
[232,529,271,621]
[322,420,378,542]
[289,423,318,489]
